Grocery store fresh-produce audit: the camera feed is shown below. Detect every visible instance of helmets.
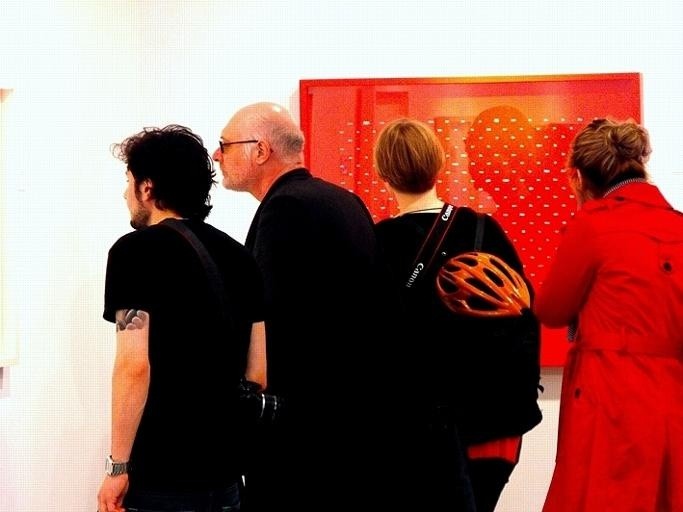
[433,249,533,320]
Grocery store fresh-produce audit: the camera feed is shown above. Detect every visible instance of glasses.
[216,135,260,153]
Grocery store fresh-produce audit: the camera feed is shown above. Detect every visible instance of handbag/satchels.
[232,377,290,436]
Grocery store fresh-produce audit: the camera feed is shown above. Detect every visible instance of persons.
[361,113,543,511]
[92,124,272,512]
[529,119,682,512]
[211,102,424,510]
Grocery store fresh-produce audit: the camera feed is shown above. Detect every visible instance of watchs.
[103,454,128,477]
